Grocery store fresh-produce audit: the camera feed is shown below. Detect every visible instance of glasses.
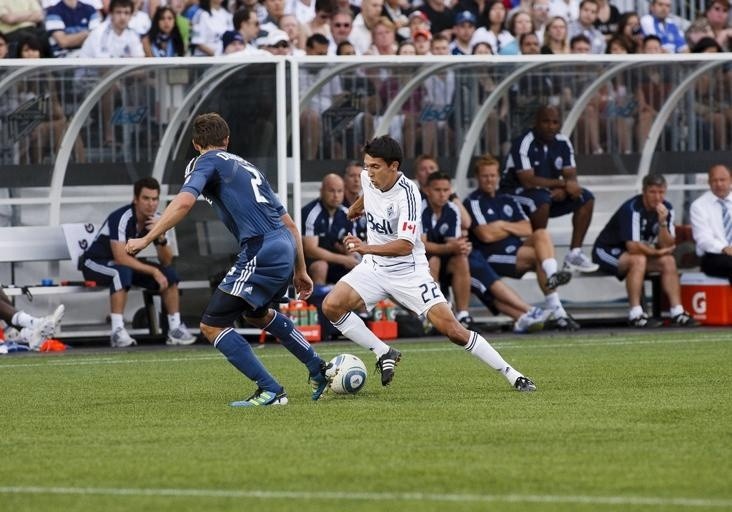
[335,23,350,28]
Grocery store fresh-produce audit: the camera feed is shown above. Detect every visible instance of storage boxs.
[678,270,732,326]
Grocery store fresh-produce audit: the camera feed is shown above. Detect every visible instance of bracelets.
[152,238,168,246]
[448,190,459,199]
[659,219,670,229]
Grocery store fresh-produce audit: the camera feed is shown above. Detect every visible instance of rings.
[349,242,354,247]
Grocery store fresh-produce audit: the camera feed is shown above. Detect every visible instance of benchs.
[448,210,685,333]
[0,219,180,341]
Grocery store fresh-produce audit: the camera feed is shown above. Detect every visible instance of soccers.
[327,352,367,393]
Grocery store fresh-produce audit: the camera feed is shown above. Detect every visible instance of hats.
[409,10,431,24]
[413,29,432,42]
[222,31,245,51]
[452,11,477,26]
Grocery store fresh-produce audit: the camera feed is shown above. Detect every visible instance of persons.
[692,163,732,282]
[416,153,553,332]
[464,156,582,331]
[0,1,731,166]
[420,172,484,333]
[321,136,533,389]
[0,282,66,352]
[125,113,327,407]
[301,174,358,332]
[508,102,600,275]
[594,174,699,328]
[346,163,365,238]
[80,179,198,346]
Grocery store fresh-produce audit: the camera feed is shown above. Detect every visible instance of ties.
[717,198,732,245]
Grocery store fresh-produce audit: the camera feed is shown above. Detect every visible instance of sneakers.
[29,333,47,351]
[374,347,401,386]
[35,304,65,335]
[307,361,339,401]
[166,322,198,346]
[111,327,138,347]
[514,250,700,333]
[512,377,536,391]
[232,386,288,406]
[459,316,481,333]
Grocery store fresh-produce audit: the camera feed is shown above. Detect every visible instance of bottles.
[373,302,396,323]
[279,303,319,327]
